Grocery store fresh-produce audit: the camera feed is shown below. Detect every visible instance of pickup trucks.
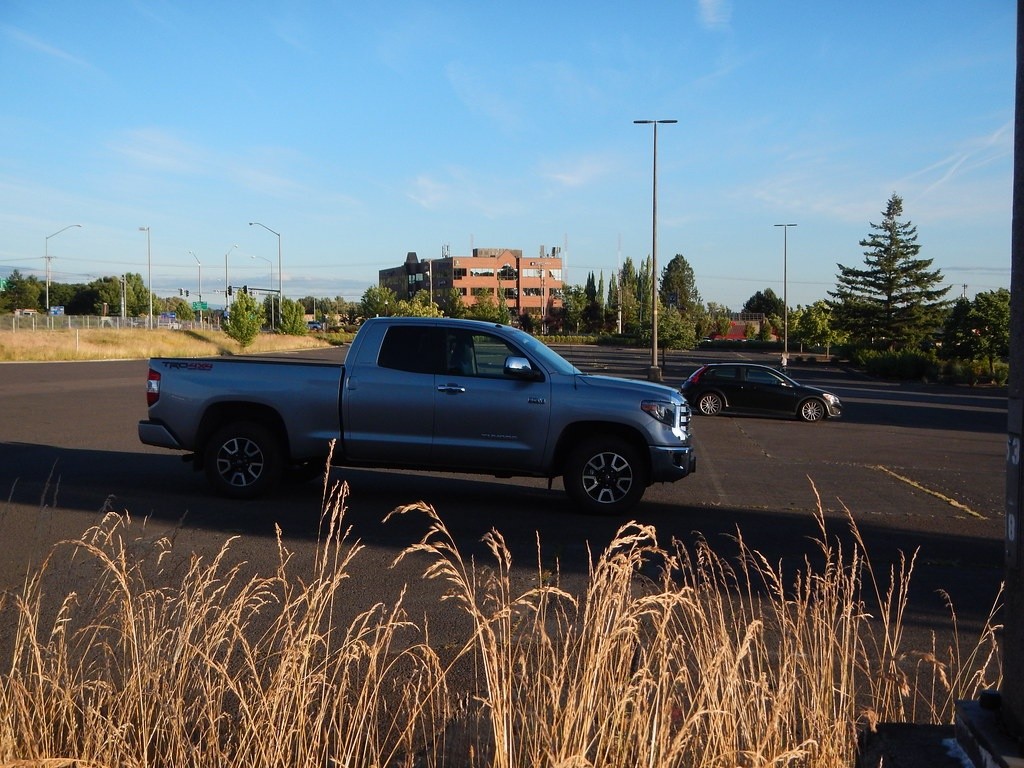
[137,316,696,514]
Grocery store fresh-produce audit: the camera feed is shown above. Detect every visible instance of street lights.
[633,119,678,382]
[249,222,282,326]
[46,224,82,315]
[774,224,798,362]
[252,256,274,329]
[139,226,153,330]
[189,251,202,323]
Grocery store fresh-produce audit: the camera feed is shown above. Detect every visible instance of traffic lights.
[225,245,238,324]
[185,290,189,297]
[180,289,183,295]
[228,286,232,295]
[242,284,247,294]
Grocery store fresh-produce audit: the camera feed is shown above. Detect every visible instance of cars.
[19,311,41,319]
[159,320,183,331]
[703,336,711,343]
[682,363,843,423]
[308,320,321,332]
